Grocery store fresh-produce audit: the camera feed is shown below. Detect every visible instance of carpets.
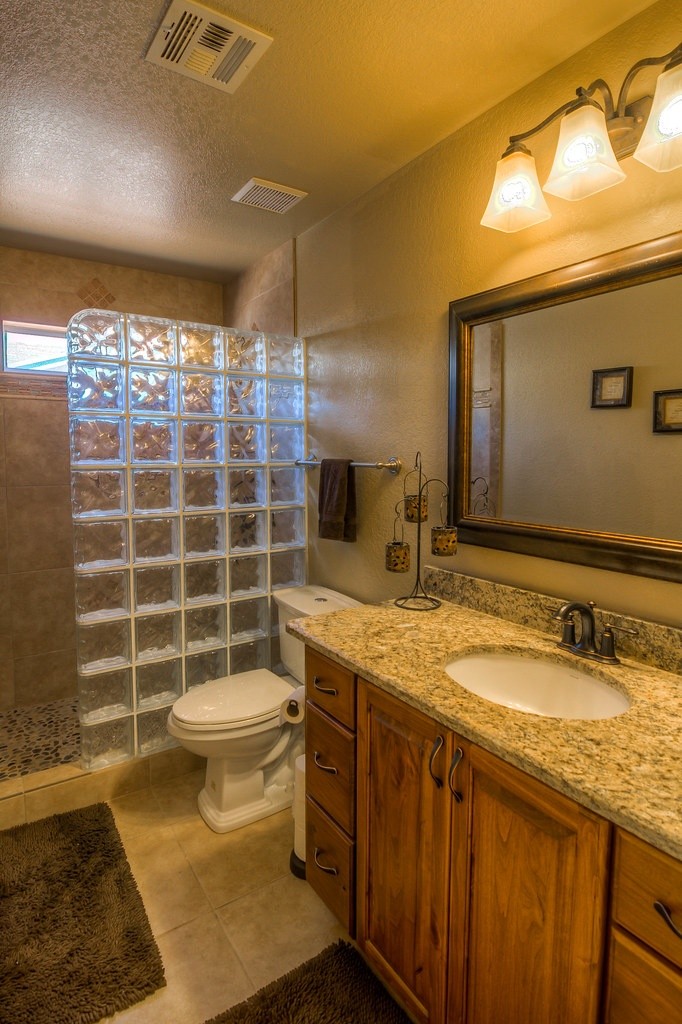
[204,937,415,1024]
[0,801,168,1024]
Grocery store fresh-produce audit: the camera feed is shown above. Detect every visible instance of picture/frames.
[590,366,634,409]
[652,388,682,433]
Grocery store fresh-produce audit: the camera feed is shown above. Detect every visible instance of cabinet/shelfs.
[303,646,682,1024]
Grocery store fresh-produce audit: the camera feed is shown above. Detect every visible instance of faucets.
[554,602,599,656]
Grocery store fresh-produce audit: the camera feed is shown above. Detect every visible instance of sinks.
[440,643,637,721]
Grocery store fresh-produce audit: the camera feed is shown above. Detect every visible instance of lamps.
[479,42,682,235]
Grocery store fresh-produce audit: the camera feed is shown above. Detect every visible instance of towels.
[317,458,358,543]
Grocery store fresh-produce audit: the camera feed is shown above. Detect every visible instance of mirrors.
[440,229,682,585]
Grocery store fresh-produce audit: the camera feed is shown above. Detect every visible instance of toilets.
[166,584,374,835]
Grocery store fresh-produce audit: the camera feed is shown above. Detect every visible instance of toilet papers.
[278,686,306,726]
